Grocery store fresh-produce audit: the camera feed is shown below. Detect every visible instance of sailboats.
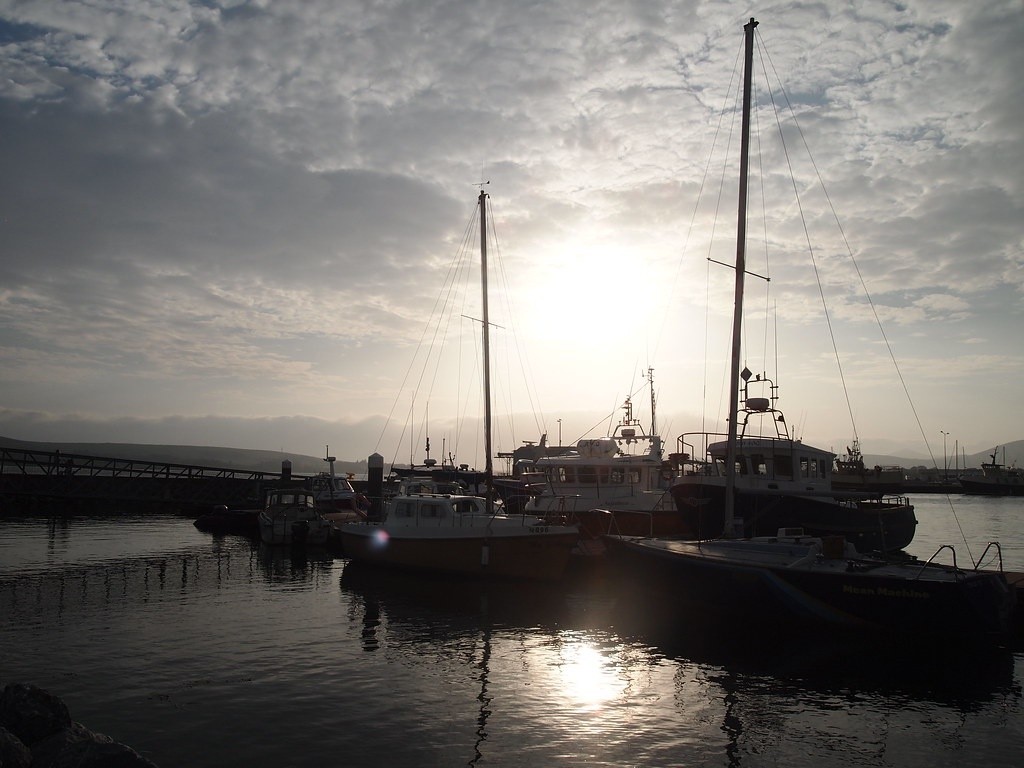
[602,13,1023,649]
[332,178,584,579]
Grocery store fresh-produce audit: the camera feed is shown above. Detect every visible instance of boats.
[520,434,696,540]
[258,488,332,551]
[302,472,355,514]
